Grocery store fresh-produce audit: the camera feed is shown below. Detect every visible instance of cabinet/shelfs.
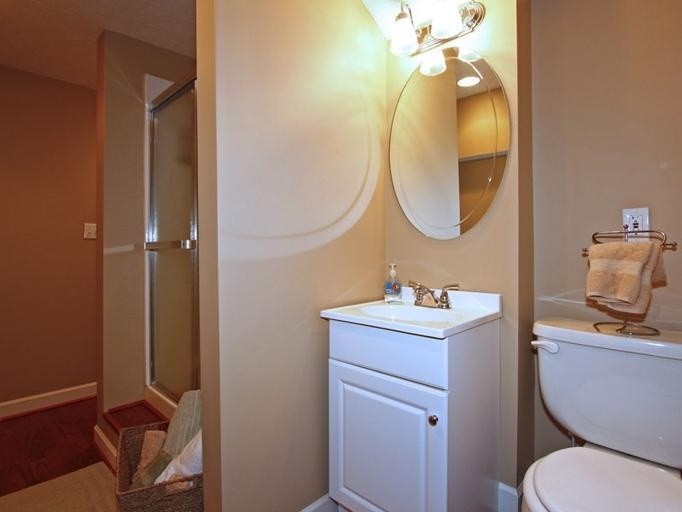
[326,320,502,512]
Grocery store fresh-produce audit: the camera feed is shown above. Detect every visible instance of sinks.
[361,305,457,323]
[414,284,439,306]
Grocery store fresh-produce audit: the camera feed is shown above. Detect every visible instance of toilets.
[520,315,682,512]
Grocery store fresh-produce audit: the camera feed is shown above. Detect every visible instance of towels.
[586,238,667,321]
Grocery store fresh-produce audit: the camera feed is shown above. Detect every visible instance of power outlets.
[622,206,649,242]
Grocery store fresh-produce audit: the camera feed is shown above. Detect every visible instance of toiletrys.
[383,263,403,305]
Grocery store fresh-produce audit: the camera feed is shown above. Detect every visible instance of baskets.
[115,419,205,512]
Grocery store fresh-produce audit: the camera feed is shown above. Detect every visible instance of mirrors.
[388,45,512,242]
